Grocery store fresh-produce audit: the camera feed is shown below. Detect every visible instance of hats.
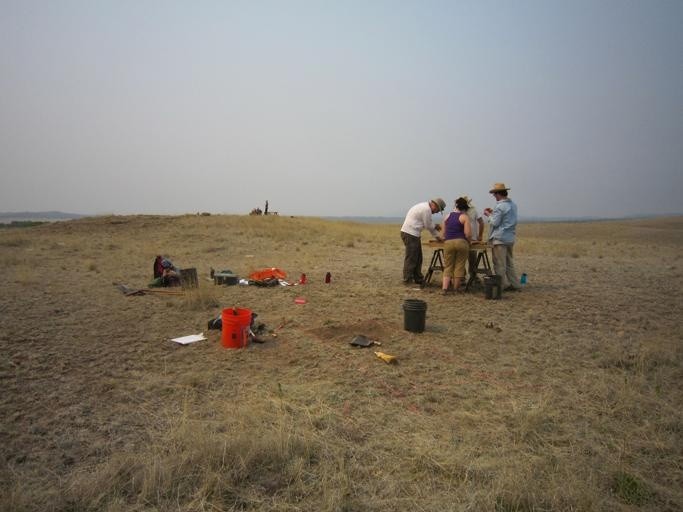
[489,182,511,193]
[431,197,446,216]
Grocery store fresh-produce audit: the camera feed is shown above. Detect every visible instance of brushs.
[374,352,397,364]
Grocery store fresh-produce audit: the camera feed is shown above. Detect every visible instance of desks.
[421,239,497,294]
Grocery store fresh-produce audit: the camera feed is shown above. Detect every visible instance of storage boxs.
[213,274,239,285]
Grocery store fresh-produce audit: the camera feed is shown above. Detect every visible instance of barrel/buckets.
[483,276,502,301]
[402,299,427,333]
[221,308,252,348]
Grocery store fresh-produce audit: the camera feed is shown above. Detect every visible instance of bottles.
[519,273,526,289]
[295,299,306,304]
[325,271,333,283]
[298,272,306,282]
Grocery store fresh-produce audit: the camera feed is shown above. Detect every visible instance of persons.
[481,184,521,292]
[450,198,484,283]
[400,197,445,286]
[441,199,474,293]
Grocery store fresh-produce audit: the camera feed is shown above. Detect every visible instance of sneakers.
[438,288,448,296]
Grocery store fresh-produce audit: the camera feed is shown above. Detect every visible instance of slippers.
[504,283,522,292]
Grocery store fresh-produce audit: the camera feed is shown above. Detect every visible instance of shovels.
[118,284,184,295]
